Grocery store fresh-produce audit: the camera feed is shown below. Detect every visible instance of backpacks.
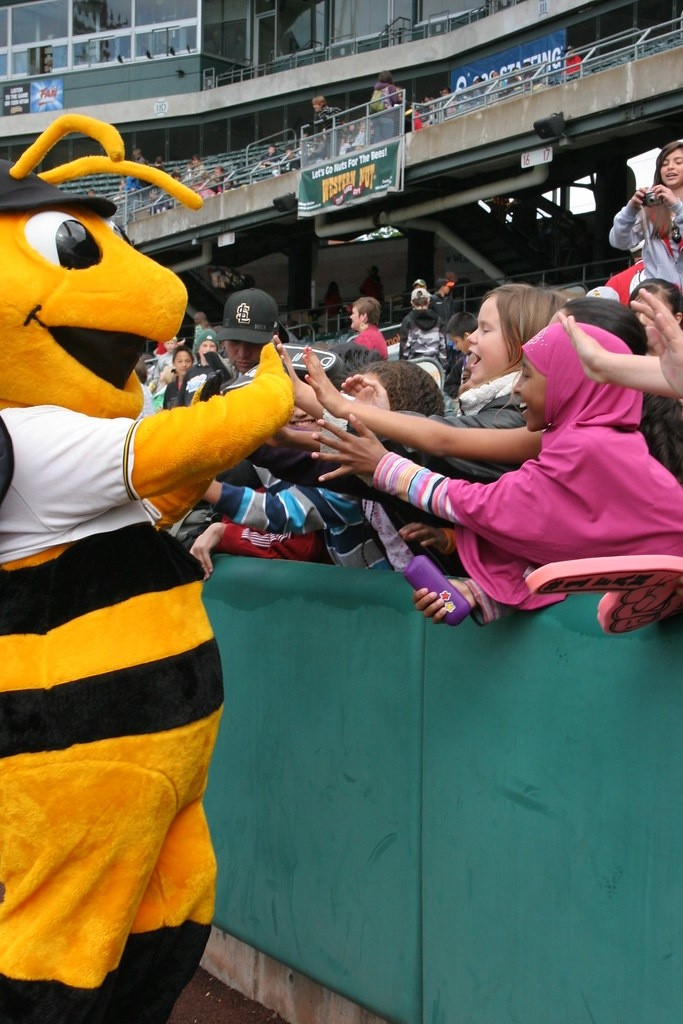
[369,87,388,118]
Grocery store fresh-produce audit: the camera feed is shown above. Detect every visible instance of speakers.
[273,194,296,213]
[533,116,566,139]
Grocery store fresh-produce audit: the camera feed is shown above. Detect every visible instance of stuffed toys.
[0,112,295,1024]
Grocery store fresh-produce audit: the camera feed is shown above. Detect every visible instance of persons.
[301,297,683,486]
[609,139,683,298]
[311,320,683,626]
[85,44,583,576]
[628,278,683,357]
[557,288,683,398]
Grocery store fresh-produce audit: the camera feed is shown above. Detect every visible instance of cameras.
[639,191,663,207]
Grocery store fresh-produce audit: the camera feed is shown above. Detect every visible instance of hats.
[196,328,218,349]
[214,288,278,344]
[412,279,426,287]
[411,288,429,301]
[436,279,455,289]
